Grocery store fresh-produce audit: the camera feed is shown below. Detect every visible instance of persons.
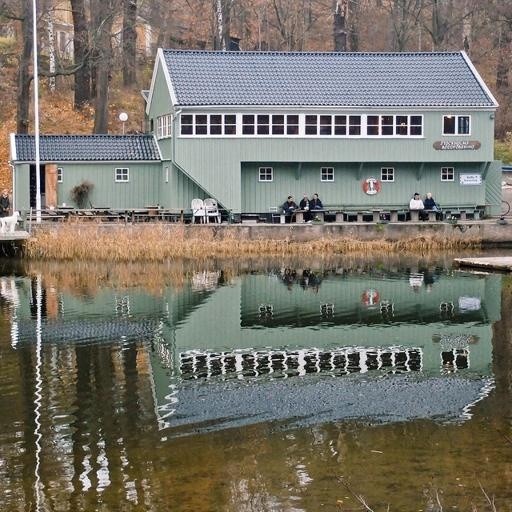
[2,277,12,321]
[282,267,325,296]
[279,196,299,223]
[408,192,425,221]
[1,188,12,234]
[421,193,438,221]
[298,192,313,223]
[423,268,443,293]
[408,269,430,294]
[309,193,323,223]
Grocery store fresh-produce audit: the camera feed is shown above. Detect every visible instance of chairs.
[190,197,222,224]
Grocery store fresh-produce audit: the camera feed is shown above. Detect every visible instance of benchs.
[31,211,180,222]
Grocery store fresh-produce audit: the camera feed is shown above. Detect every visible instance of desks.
[56,206,168,213]
[290,208,485,224]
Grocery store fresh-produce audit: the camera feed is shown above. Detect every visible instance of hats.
[415,193,419,195]
